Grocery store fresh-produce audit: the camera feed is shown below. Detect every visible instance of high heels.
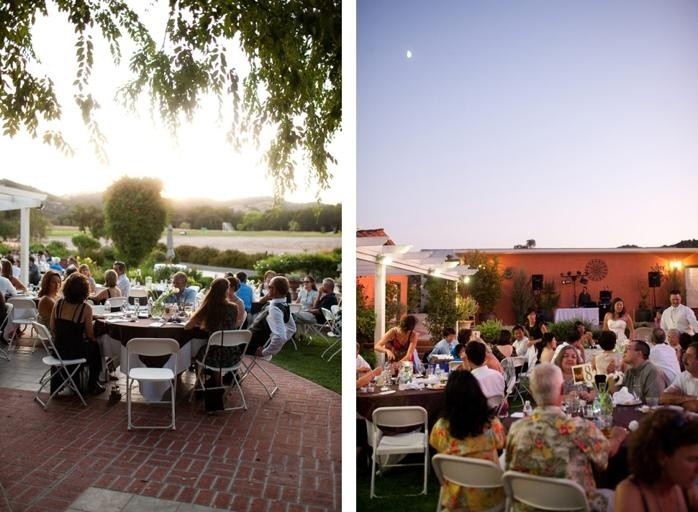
[87,382,105,396]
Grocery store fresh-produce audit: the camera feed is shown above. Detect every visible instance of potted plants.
[457,296,479,329]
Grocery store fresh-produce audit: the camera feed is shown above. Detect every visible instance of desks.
[554,307,600,326]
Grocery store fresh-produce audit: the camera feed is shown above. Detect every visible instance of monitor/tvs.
[600,291,611,302]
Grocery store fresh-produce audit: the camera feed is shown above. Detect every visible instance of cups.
[144,276,153,291]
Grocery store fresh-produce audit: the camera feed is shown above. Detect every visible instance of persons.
[506,364,631,511]
[611,405,697,511]
[1,250,343,354]
[357,343,386,475]
[49,272,107,401]
[184,275,241,396]
[430,368,507,512]
[249,275,297,358]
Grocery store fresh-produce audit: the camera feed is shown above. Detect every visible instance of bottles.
[577,404,594,417]
[425,364,440,378]
[104,300,112,313]
[522,400,533,418]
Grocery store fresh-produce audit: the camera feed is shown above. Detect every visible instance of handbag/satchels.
[203,388,225,412]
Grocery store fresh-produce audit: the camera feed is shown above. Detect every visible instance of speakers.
[649,272,660,287]
[532,274,543,290]
